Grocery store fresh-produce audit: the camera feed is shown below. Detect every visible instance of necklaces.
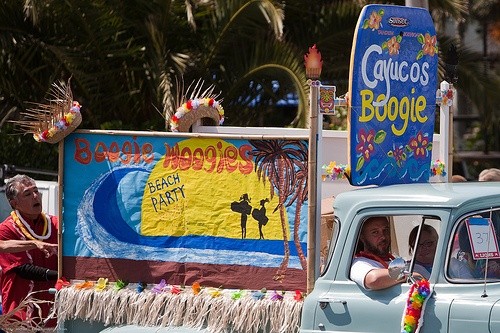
[359,251,395,270]
[10,210,51,242]
[462,253,484,278]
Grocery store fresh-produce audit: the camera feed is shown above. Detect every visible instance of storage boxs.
[320,192,337,263]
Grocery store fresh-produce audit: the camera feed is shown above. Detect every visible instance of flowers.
[321,160,447,182]
[401,279,434,333]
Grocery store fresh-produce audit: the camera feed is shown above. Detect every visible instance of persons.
[457,224,499,279]
[0,174,59,329]
[409,225,439,282]
[349,217,425,291]
[0,239,58,258]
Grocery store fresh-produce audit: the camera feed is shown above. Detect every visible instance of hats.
[452,175,467,182]
[479,168,500,181]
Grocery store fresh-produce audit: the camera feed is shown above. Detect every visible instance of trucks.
[57,182,500,333]
